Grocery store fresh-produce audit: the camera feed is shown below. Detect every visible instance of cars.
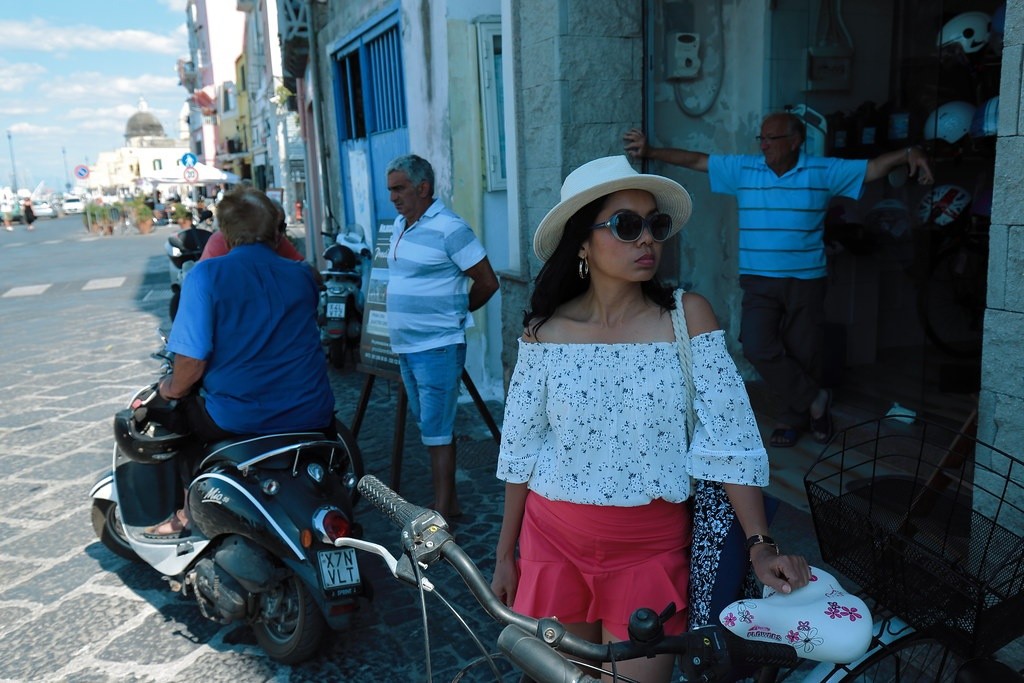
[31,200,58,218]
[62,197,86,215]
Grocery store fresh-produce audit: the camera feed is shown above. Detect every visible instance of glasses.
[755,133,794,143]
[591,209,673,243]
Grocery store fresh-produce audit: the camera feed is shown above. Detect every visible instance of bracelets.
[745,534,780,562]
[905,144,914,153]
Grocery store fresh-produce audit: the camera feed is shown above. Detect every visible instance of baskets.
[805,414,1024,654]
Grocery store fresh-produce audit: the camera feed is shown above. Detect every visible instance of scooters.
[319,197,371,368]
[88,327,374,660]
[165,211,214,322]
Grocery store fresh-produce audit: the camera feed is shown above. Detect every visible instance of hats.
[533,154,692,263]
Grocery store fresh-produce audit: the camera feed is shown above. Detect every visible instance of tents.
[148,161,228,222]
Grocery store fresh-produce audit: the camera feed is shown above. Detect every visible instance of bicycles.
[331,414,1023,683]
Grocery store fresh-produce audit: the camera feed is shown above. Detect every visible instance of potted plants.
[83,196,191,236]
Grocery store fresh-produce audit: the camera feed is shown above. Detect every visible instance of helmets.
[937,12,992,52]
[917,185,970,225]
[993,5,1007,33]
[925,98,974,145]
[114,409,194,464]
[970,95,998,136]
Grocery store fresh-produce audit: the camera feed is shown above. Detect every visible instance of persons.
[199,200,326,284]
[491,154,813,683]
[144,184,335,539]
[385,155,500,533]
[0,194,38,231]
[623,112,937,447]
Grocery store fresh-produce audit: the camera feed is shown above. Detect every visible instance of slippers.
[143,509,192,538]
[810,390,833,443]
[769,422,800,448]
